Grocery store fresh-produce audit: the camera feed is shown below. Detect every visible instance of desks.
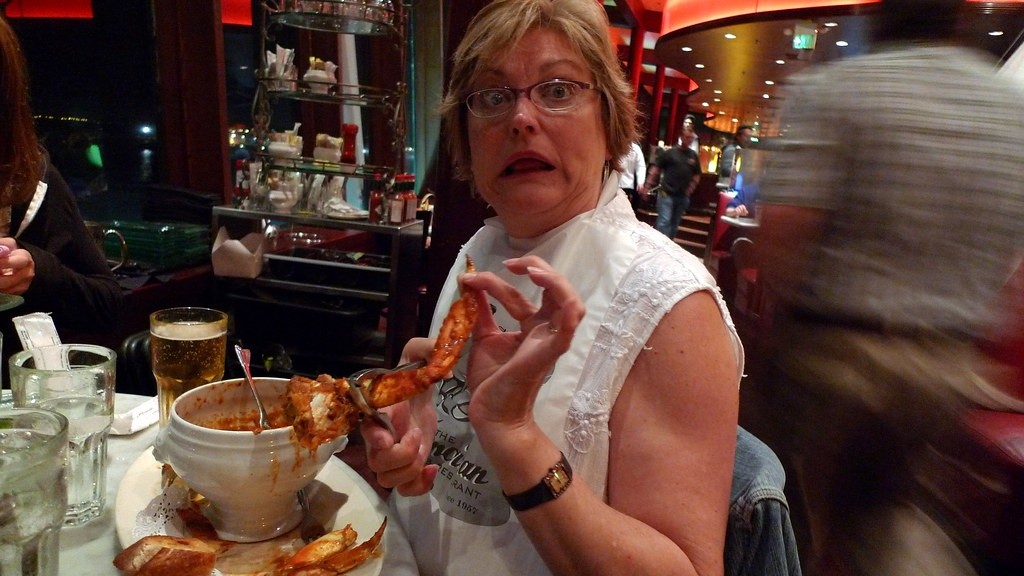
[1,388,422,576]
[719,215,761,230]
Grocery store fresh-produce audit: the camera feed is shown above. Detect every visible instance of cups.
[9,344,117,531]
[150,306,229,431]
[0,408,69,576]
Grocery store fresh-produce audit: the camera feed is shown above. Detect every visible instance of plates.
[115,443,385,576]
[327,213,369,219]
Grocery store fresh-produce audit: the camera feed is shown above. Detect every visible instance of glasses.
[459,79,602,120]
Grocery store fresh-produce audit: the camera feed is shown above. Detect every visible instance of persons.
[754,0,1024,576]
[356,1,746,576]
[0,17,125,389]
[616,111,789,240]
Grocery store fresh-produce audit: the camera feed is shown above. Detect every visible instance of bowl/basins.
[166,378,340,545]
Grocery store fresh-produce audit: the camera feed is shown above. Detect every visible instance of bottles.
[368,173,417,226]
[229,125,255,201]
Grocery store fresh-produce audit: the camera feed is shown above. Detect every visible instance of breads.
[112,529,217,576]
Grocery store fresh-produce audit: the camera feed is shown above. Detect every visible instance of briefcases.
[101,216,211,271]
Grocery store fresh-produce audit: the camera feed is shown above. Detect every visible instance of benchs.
[705,189,756,317]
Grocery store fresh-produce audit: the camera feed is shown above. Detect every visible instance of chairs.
[723,426,802,576]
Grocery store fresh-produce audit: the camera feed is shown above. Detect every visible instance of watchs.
[502,451,573,512]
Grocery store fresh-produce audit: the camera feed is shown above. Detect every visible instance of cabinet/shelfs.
[234,0,412,221]
[209,204,424,380]
